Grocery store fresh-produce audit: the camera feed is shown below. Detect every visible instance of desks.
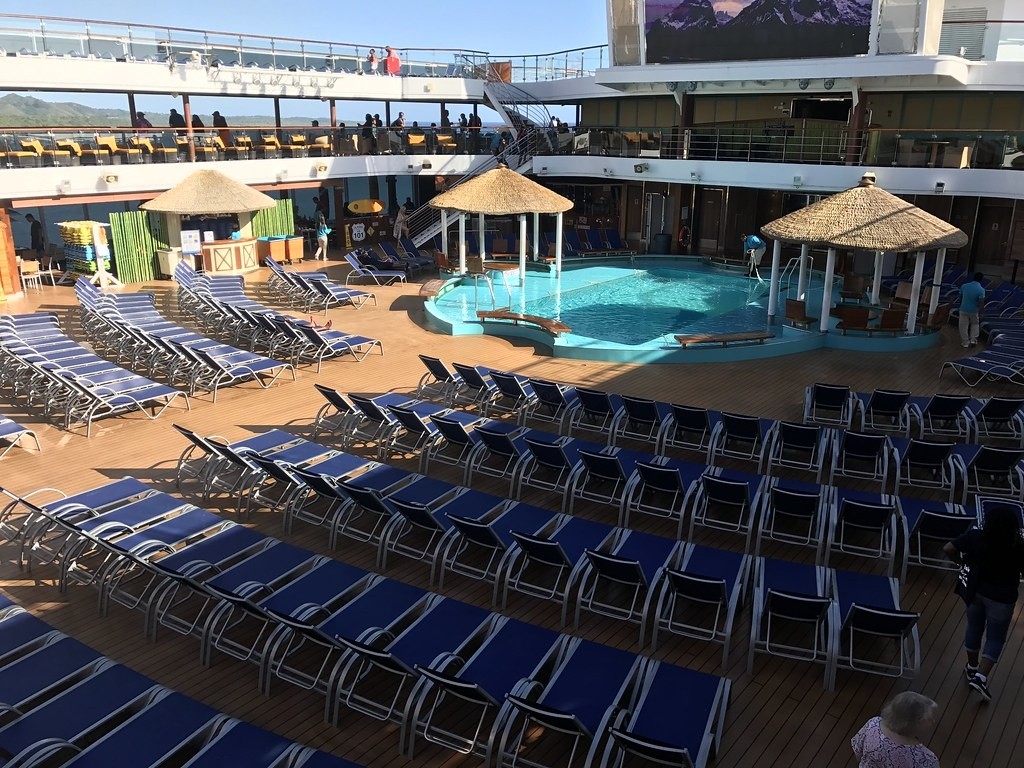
[483,261,520,278]
[920,140,950,166]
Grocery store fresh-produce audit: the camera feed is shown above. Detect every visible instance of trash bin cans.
[277,235,304,265]
[257,236,286,266]
[654,234,672,255]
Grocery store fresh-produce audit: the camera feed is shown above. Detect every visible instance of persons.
[958,272,986,347]
[681,225,689,247]
[441,178,450,193]
[740,234,767,277]
[212,111,230,132]
[358,197,417,271]
[850,691,940,768]
[227,222,240,239]
[24,213,45,253]
[310,109,570,168]
[311,197,333,262]
[287,316,332,332]
[366,49,379,74]
[342,202,355,218]
[169,109,187,136]
[135,112,152,137]
[943,507,1024,701]
[381,46,401,75]
[191,115,205,134]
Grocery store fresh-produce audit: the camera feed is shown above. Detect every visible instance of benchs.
[476,310,572,338]
[675,331,776,349]
[419,278,446,300]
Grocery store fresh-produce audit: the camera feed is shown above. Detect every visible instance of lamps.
[542,166,548,174]
[233,74,342,85]
[666,82,677,91]
[799,79,811,90]
[824,78,834,90]
[794,175,803,187]
[934,182,945,193]
[602,168,611,175]
[687,81,698,91]
[691,172,700,181]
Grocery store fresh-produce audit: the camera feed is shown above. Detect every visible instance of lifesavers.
[679,226,690,247]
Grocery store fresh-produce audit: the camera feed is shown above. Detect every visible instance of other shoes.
[971,341,978,344]
[315,253,319,260]
[960,343,968,348]
[323,258,329,261]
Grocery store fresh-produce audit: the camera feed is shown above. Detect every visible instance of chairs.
[622,131,664,151]
[942,139,978,167]
[999,135,1024,168]
[0,131,496,170]
[0,46,470,78]
[898,139,927,166]
[0,221,1024,768]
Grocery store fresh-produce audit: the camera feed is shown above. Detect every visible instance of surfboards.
[348,199,386,213]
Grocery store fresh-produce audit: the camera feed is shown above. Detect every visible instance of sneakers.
[970,676,993,700]
[963,662,980,681]
